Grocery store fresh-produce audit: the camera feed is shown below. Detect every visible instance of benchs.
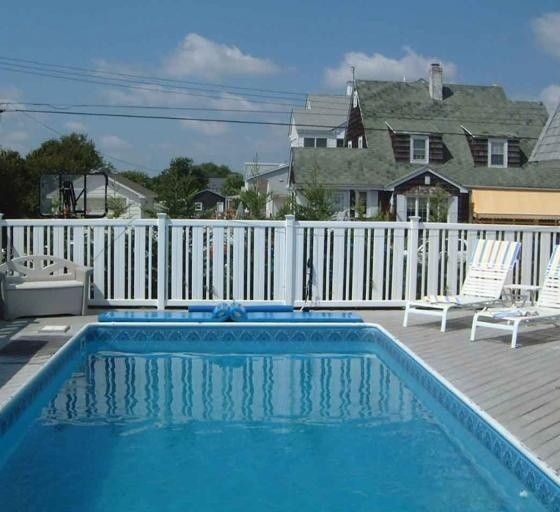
[0,255,92,321]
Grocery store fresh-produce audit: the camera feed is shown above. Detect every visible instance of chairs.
[403,240,522,332]
[469,245,560,348]
